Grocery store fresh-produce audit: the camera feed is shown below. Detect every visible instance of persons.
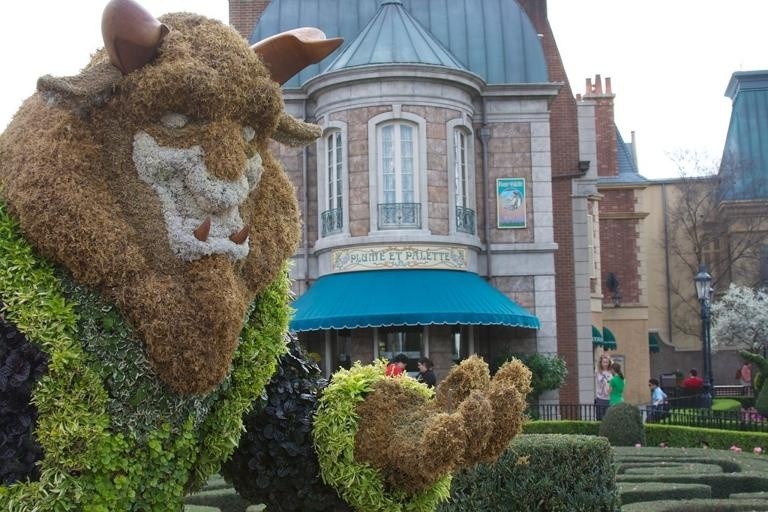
[682,368,704,387]
[415,356,437,387]
[648,377,669,408]
[735,362,753,386]
[386,353,410,376]
[593,353,626,419]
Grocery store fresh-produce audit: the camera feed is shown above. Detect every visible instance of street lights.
[693,259,715,405]
